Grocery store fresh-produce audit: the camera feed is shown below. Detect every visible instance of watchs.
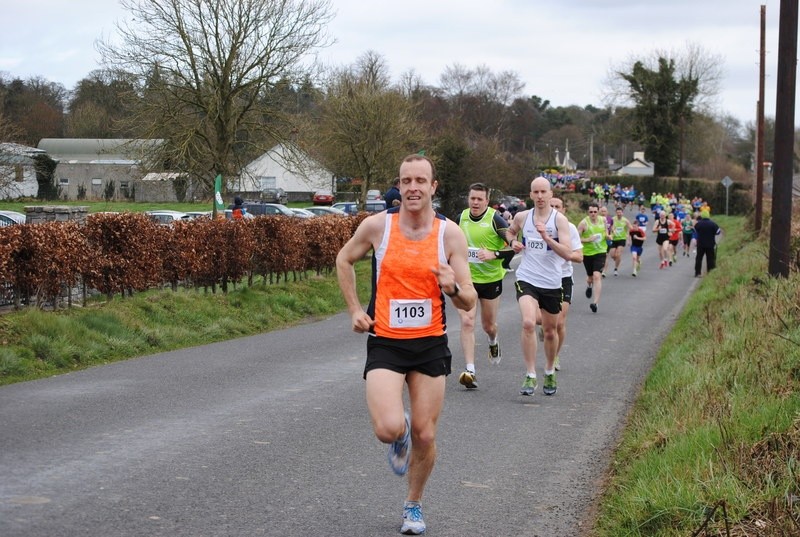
[494,250,500,260]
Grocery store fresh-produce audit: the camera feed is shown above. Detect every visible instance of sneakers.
[519,370,539,395]
[459,367,478,389]
[388,413,413,476]
[543,367,558,394]
[399,500,425,535]
[488,336,502,366]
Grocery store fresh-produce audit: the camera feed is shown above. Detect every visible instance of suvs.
[228,202,296,218]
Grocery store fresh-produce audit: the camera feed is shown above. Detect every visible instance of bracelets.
[442,282,460,297]
[509,239,517,247]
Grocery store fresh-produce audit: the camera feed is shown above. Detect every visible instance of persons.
[506,177,573,396]
[384,177,402,209]
[454,183,519,389]
[489,200,522,272]
[536,172,720,372]
[336,154,478,535]
[235,191,244,209]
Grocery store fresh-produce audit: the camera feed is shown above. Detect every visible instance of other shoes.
[632,271,637,277]
[590,303,597,313]
[553,356,562,370]
[602,273,607,278]
[539,325,546,343]
[613,270,619,275]
[586,283,591,298]
[659,251,690,268]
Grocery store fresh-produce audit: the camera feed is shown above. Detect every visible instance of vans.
[329,200,387,215]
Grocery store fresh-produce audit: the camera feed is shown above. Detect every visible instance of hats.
[700,209,710,219]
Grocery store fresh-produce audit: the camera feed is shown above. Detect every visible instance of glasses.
[589,211,598,214]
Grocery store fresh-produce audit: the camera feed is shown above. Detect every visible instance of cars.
[276,208,315,218]
[260,188,289,206]
[0,210,26,228]
[145,209,254,230]
[498,196,526,212]
[313,189,335,206]
[303,206,349,217]
[366,189,385,200]
